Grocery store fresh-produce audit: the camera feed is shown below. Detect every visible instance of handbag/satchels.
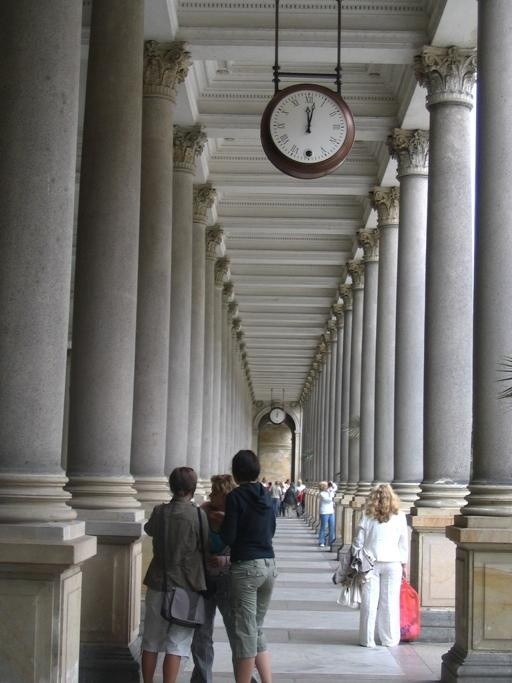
[399,578,421,643]
[160,586,205,628]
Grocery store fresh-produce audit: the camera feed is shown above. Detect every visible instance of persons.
[219,447,278,682]
[190,473,258,682]
[260,476,306,518]
[142,466,211,682]
[348,483,409,649]
[316,480,338,547]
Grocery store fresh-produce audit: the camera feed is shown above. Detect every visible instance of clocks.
[260,1,355,180]
[270,388,286,425]
